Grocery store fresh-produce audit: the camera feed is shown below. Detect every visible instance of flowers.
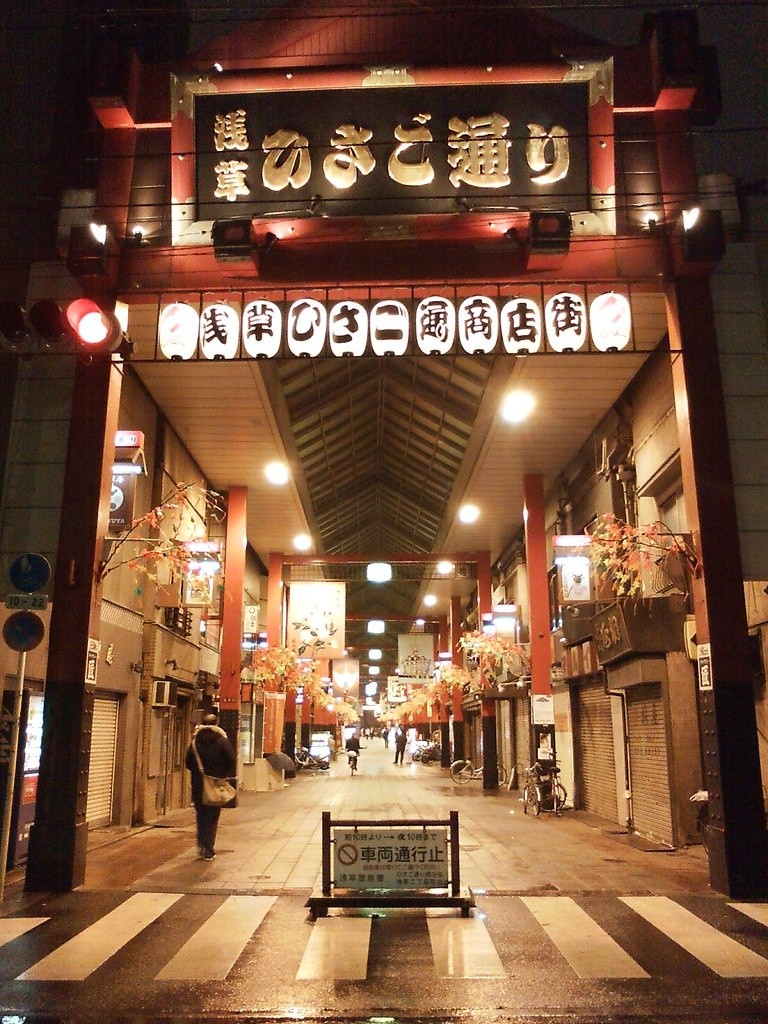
[580,513,696,612]
[100,481,230,609]
[376,630,536,724]
[241,646,361,725]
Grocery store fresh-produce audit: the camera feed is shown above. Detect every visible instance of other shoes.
[198,844,216,860]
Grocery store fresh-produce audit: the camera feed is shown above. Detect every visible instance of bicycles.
[539,752,567,817]
[523,762,542,816]
[449,754,508,787]
[348,746,361,776]
[412,743,442,765]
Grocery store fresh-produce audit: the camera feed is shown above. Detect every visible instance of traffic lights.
[0,296,122,354]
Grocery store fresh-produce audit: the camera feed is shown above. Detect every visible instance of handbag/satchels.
[202,774,237,807]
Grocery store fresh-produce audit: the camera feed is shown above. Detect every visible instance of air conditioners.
[152,680,178,708]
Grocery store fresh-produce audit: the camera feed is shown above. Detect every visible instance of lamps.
[505,228,527,252]
[648,220,660,240]
[257,232,277,256]
[131,229,147,248]
[227,195,324,219]
[454,196,531,213]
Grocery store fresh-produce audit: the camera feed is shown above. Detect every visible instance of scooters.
[295,747,330,771]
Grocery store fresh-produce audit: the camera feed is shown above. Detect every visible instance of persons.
[365,727,374,740]
[185,714,237,863]
[392,727,407,765]
[383,727,389,748]
[346,732,360,771]
